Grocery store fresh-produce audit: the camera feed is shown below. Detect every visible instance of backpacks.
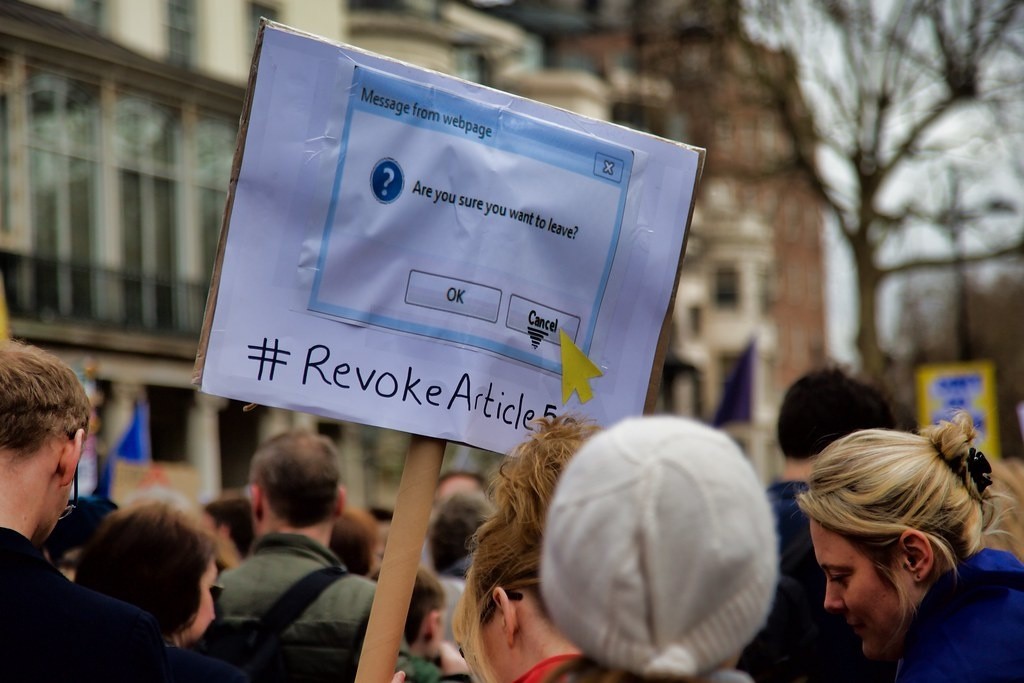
[191,564,350,683]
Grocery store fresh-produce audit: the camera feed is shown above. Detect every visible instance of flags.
[714,345,753,426]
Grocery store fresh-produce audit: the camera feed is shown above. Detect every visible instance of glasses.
[195,576,224,602]
[59,430,78,522]
[457,590,522,658]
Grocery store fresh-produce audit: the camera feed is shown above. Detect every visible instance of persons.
[541,416,779,683]
[736,370,897,683]
[44,432,496,683]
[795,413,1023,683]
[1,340,166,683]
[454,412,604,683]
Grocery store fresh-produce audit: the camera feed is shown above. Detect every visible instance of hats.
[536,412,781,678]
